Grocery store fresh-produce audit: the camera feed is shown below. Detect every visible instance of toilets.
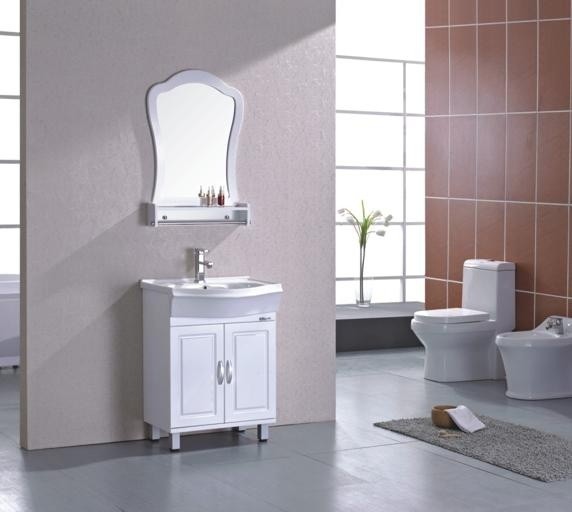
[410,259,517,382]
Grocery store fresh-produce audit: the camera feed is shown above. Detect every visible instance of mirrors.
[144,68,245,207]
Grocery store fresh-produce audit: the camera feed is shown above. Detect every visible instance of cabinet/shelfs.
[141,311,277,428]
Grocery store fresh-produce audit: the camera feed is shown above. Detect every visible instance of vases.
[353,259,374,308]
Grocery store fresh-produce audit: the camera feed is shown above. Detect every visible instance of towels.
[443,403,486,433]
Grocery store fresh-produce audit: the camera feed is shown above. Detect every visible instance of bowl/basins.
[431,405,457,428]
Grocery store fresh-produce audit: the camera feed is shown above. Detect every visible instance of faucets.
[194,248,214,284]
[544,316,564,335]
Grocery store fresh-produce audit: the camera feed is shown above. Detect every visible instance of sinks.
[494,314,572,400]
[159,281,264,289]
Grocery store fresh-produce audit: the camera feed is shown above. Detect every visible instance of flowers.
[337,198,393,303]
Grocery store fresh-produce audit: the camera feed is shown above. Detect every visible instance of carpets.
[372,409,572,484]
